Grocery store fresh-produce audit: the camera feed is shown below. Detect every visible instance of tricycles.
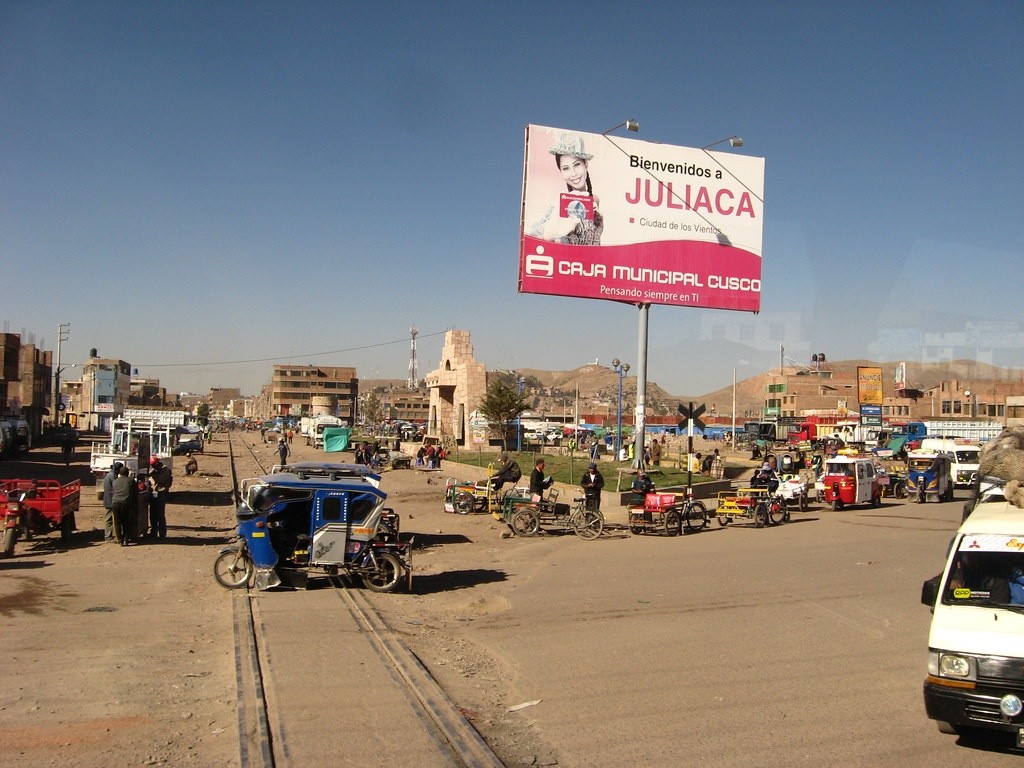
[444,464,531,515]
[773,474,824,509]
[493,488,605,541]
[621,486,711,537]
[715,479,791,527]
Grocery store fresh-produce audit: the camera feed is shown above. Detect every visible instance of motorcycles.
[0,478,80,557]
[212,472,414,592]
[178,429,204,455]
[271,463,371,481]
[814,449,953,511]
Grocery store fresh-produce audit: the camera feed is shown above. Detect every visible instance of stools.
[427,459,433,469]
[415,458,423,466]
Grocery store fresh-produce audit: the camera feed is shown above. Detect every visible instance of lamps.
[702,136,743,149]
[603,118,640,136]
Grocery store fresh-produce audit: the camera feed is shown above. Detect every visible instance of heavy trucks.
[891,420,1002,447]
[788,422,883,451]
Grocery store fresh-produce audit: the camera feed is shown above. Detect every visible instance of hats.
[760,462,771,470]
[549,135,595,161]
[498,459,502,461]
[638,469,646,475]
[587,463,597,468]
[437,445,441,447]
[363,441,371,444]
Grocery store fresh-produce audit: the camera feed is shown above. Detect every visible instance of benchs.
[634,494,675,512]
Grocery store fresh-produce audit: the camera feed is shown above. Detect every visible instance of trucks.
[889,422,928,441]
[90,415,173,501]
[300,415,342,450]
[921,472,1024,752]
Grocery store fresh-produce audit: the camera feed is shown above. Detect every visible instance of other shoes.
[116,537,123,541]
[123,540,137,545]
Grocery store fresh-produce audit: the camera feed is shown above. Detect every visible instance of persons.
[58,430,795,546]
[550,134,603,246]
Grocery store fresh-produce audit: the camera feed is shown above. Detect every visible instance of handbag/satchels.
[440,451,446,459]
[543,476,554,486]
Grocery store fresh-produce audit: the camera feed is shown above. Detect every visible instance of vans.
[921,438,981,488]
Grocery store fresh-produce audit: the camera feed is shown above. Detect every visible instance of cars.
[523,424,593,441]
[380,420,428,437]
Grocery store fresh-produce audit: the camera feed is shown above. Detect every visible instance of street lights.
[53,322,77,427]
[612,359,630,462]
[514,373,526,456]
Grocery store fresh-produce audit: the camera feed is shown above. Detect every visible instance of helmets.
[149,456,159,464]
